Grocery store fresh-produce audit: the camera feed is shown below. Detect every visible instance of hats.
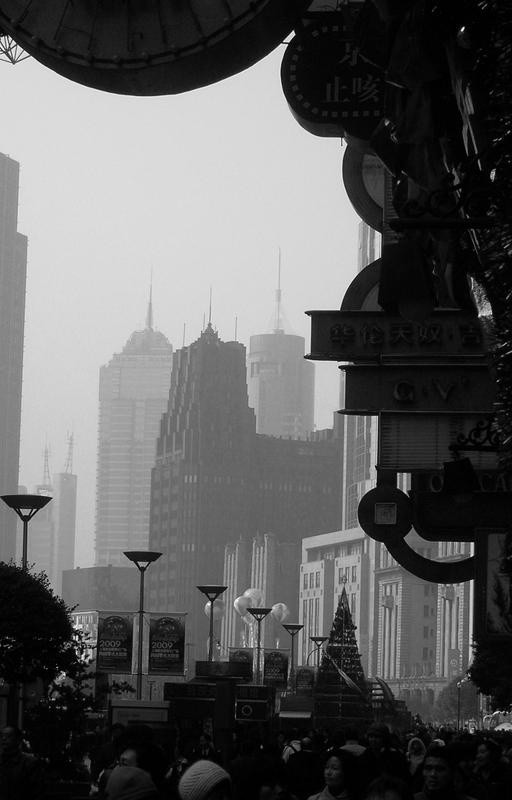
[178,759,231,798]
[106,765,156,799]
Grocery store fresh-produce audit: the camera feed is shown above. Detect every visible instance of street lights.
[119,549,162,698]
[244,606,275,684]
[280,621,307,690]
[454,681,464,736]
[195,583,229,673]
[305,636,329,670]
[0,493,54,793]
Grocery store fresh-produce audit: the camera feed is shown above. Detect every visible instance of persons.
[2,714,511,799]
